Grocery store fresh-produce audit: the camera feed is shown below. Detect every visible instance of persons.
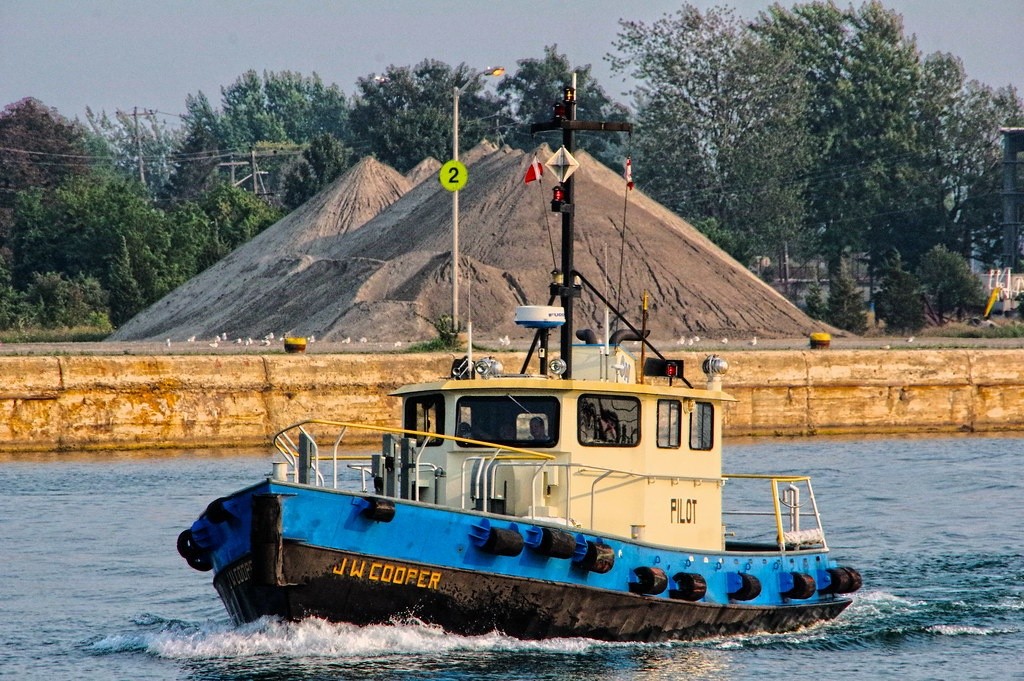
[530,417,549,440]
[458,422,475,440]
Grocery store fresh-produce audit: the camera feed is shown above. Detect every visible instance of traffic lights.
[552,186,564,202]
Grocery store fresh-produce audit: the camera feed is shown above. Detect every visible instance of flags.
[525,155,543,182]
[624,158,634,191]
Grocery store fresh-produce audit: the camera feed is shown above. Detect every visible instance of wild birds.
[499,334,510,345]
[906,336,915,342]
[165,331,402,349]
[677,336,757,346]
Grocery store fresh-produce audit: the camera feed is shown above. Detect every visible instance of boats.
[178,86,863,643]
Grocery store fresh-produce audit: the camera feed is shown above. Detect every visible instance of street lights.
[453,66,505,332]
